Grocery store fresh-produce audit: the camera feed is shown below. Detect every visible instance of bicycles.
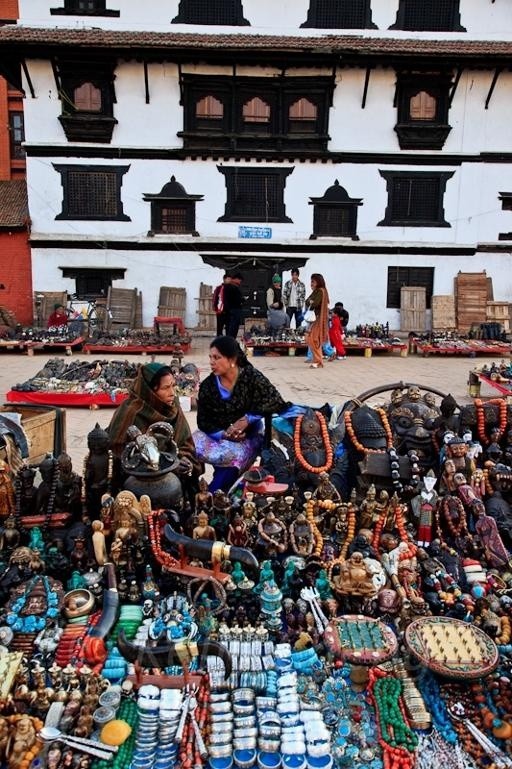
[60,294,113,333]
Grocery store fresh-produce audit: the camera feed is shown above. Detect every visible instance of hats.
[272,274,281,283]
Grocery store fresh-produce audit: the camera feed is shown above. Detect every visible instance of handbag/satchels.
[304,306,317,322]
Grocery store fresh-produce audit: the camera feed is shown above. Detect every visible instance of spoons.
[447,700,510,768]
[34,727,119,761]
[300,588,323,633]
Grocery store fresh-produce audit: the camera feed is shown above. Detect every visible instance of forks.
[306,586,330,626]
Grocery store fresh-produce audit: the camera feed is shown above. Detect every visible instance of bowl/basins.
[62,588,95,619]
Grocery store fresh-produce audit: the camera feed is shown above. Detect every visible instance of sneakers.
[304,355,346,368]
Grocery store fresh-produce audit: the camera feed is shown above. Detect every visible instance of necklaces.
[344,409,393,453]
[436,496,471,561]
[372,504,418,563]
[444,499,467,538]
[293,411,334,474]
[305,496,356,569]
[441,674,512,769]
[388,447,421,492]
[368,664,418,769]
[14,458,60,536]
[146,510,177,569]
[0,713,44,769]
[474,397,508,442]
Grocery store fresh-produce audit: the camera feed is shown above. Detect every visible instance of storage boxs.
[0,405,67,464]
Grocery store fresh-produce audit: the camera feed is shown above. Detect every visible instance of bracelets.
[133,642,334,769]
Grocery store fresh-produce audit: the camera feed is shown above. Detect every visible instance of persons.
[471,498,512,574]
[106,364,195,466]
[440,428,512,505]
[211,265,349,368]
[192,480,313,557]
[47,304,68,329]
[197,339,286,497]
[71,491,152,567]
